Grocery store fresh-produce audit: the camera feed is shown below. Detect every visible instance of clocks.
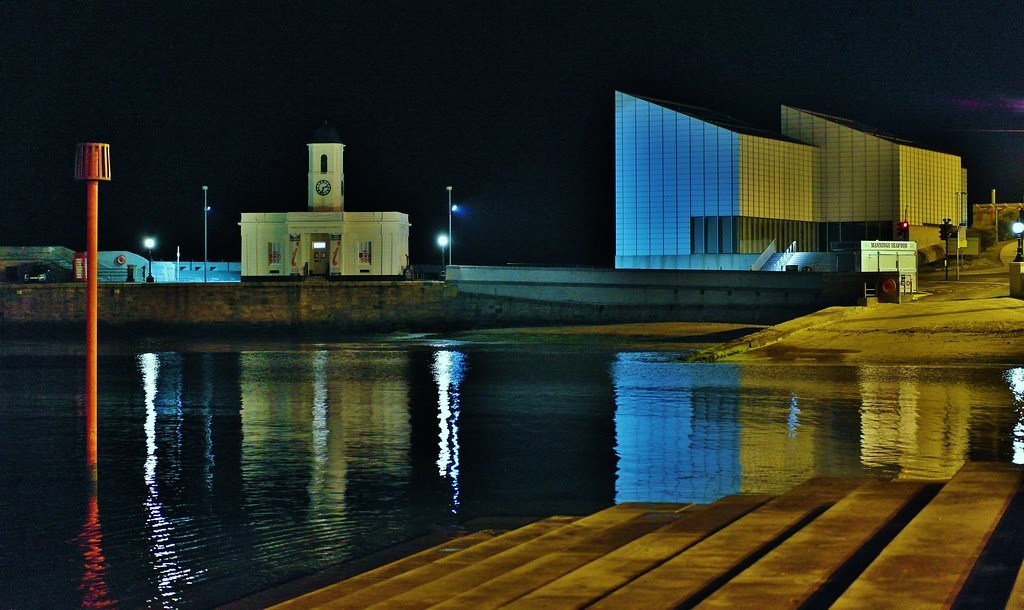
[316,180,331,196]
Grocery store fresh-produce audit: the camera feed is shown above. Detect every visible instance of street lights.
[144,239,155,282]
[436,233,449,280]
[202,185,209,284]
[961,192,970,225]
[1012,217,1024,262]
[446,186,454,268]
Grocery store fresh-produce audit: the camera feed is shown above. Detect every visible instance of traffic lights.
[939,225,945,240]
[896,222,902,236]
[901,221,908,230]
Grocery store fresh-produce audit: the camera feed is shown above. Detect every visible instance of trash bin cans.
[404,269,415,280]
[126,264,137,282]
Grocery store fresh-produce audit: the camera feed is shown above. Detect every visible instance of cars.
[22,262,57,284]
